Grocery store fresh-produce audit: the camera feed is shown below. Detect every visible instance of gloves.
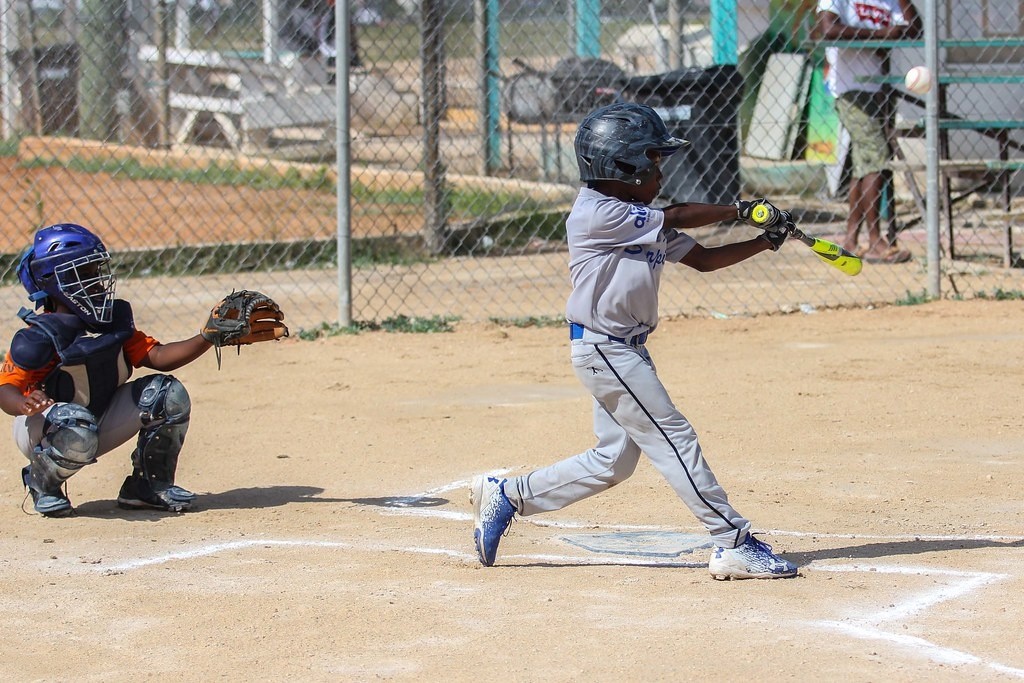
[760,210,794,251]
[734,199,782,233]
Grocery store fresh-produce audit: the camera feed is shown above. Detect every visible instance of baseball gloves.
[199,287,291,348]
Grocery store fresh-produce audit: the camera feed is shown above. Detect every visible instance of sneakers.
[469,474,518,566]
[709,531,797,580]
[22,481,73,518]
[116,476,195,511]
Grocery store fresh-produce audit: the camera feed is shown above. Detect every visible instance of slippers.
[843,244,867,260]
[867,247,911,264]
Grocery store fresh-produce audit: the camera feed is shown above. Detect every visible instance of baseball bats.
[751,203,863,277]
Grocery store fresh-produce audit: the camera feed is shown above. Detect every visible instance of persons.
[815,0,925,264]
[471,105,798,582]
[0,223,289,516]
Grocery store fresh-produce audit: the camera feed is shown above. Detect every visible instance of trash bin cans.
[4,42,81,135]
[630,65,742,224]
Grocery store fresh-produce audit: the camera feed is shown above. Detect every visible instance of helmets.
[17,223,116,327]
[573,102,691,186]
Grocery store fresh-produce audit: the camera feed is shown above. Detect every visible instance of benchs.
[114,74,358,153]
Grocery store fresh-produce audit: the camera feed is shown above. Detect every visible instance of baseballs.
[904,65,934,95]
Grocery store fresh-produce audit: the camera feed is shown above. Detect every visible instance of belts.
[569,323,650,347]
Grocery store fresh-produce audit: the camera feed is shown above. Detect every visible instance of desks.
[136,45,305,139]
